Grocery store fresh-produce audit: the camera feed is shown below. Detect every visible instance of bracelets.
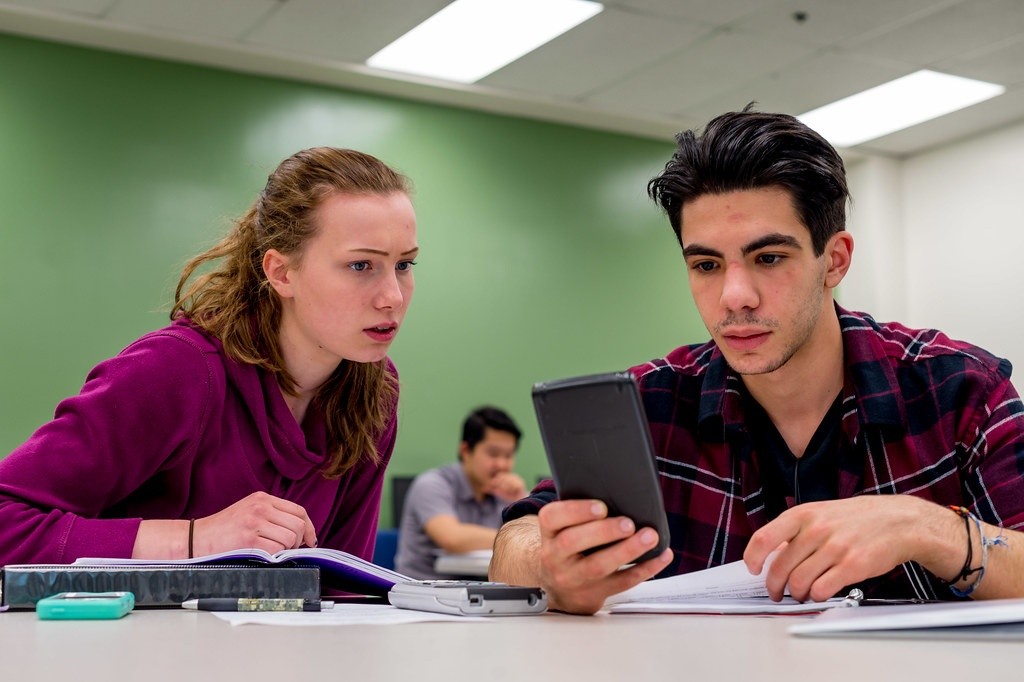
[189,519,195,559]
[950,505,1007,598]
[943,509,986,587]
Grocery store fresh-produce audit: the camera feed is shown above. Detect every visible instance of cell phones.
[532,370,670,564]
[388,580,548,616]
[35,592,136,620]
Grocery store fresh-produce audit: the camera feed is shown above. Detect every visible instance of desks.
[0,614,1024,682]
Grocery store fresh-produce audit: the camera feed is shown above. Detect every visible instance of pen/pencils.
[179,597,335,616]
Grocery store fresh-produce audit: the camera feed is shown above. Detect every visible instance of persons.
[0,146,418,603]
[488,99,1023,616]
[394,406,528,580]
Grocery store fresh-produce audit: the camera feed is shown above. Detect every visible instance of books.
[79,546,1024,636]
